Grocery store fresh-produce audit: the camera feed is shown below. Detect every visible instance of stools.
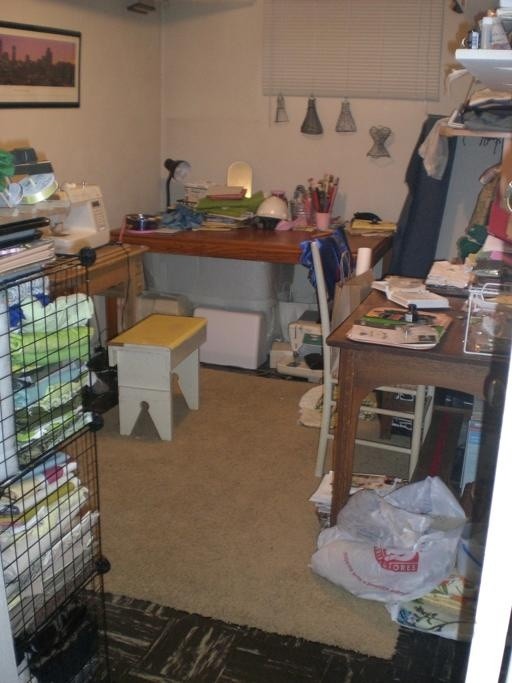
[106,313,207,440]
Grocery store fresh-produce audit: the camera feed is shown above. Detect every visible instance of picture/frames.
[0,20,83,109]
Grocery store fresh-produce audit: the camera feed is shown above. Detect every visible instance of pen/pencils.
[308,175,341,213]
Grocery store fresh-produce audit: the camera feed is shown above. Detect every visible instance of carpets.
[84,579,469,683]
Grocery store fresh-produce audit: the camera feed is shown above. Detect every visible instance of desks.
[47,243,150,416]
[325,258,512,526]
[110,212,398,355]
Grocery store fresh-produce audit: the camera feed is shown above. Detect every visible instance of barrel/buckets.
[456,522,486,583]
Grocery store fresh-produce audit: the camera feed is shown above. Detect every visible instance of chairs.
[309,223,436,483]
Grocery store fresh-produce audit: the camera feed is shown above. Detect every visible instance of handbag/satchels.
[330,250,375,380]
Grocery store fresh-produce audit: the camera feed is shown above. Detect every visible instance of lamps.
[163,156,192,212]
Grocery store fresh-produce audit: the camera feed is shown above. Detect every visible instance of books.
[309,471,409,520]
[184,181,254,231]
